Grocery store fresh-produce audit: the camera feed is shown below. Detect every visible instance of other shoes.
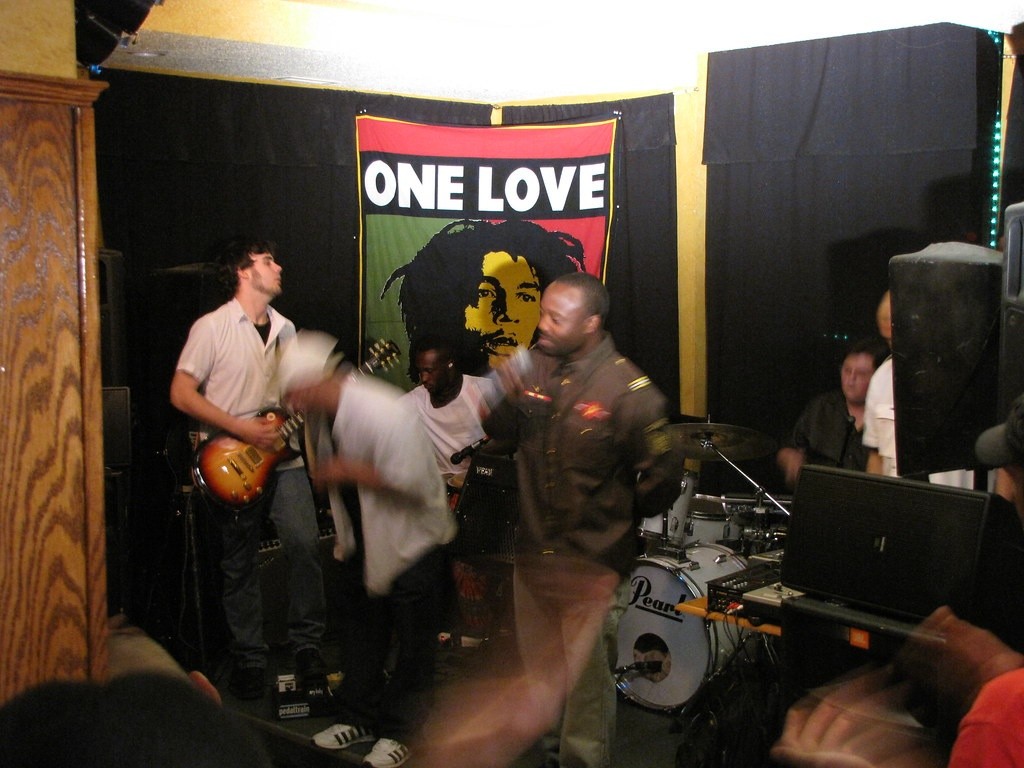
[231,664,266,702]
[296,646,322,680]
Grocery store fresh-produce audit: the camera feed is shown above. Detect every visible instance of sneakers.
[361,738,412,767]
[310,721,379,750]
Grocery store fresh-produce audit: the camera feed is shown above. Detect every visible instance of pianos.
[696,487,793,516]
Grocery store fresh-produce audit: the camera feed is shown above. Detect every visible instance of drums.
[612,540,762,714]
[642,492,757,551]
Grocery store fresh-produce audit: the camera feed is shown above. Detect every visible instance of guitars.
[191,337,403,515]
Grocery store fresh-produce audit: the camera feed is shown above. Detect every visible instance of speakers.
[779,201,1023,633]
[100,387,135,619]
[443,455,518,566]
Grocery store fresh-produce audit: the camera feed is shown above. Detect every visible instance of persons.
[862,289,1024,517]
[771,604,1024,768]
[279,330,457,768]
[170,240,326,710]
[478,272,681,768]
[777,340,885,483]
[402,339,494,474]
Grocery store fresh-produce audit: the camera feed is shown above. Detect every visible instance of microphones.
[449,435,491,465]
[627,661,662,672]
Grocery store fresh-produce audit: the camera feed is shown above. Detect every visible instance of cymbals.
[665,421,780,462]
